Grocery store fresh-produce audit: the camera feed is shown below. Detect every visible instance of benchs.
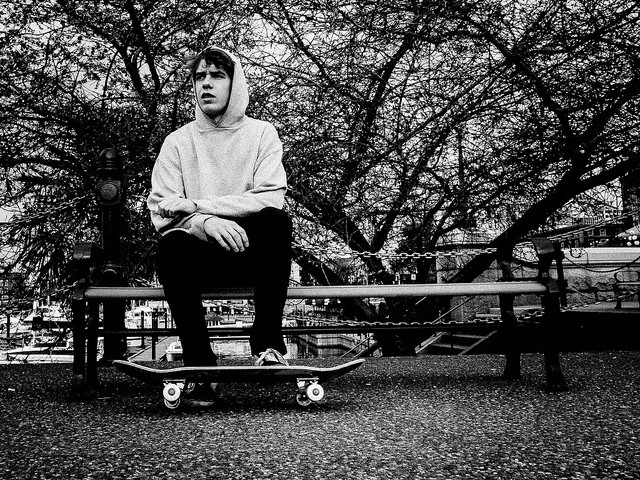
[70,242,570,394]
[612,268,639,310]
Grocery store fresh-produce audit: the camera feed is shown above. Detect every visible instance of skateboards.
[112,359,365,411]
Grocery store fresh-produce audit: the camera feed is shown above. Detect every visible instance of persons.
[145,45,292,407]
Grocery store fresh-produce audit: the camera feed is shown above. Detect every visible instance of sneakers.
[188,383,217,407]
[254,349,289,366]
